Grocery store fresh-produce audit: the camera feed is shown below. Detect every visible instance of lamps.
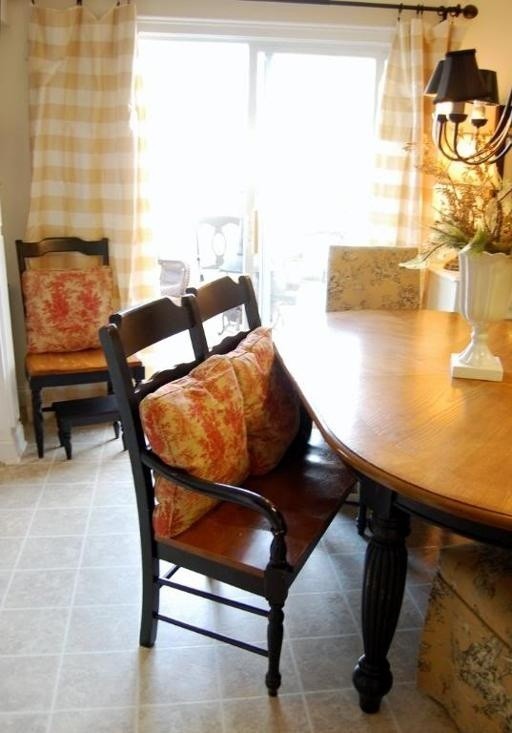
[440,132,501,270]
[423,48,512,164]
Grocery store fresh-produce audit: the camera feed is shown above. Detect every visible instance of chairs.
[14,238,145,458]
[326,246,419,310]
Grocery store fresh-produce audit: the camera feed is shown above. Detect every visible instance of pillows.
[222,323,312,475]
[21,262,121,354]
[140,352,252,540]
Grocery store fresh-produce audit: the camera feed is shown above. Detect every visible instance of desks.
[271,311,512,714]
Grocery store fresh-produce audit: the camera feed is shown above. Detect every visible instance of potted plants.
[398,128,512,382]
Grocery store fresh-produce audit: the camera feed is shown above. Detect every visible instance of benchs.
[97,275,370,699]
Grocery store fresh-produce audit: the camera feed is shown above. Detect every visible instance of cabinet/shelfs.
[422,264,462,311]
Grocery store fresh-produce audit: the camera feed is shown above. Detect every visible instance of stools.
[415,544,512,733]
[51,395,130,460]
